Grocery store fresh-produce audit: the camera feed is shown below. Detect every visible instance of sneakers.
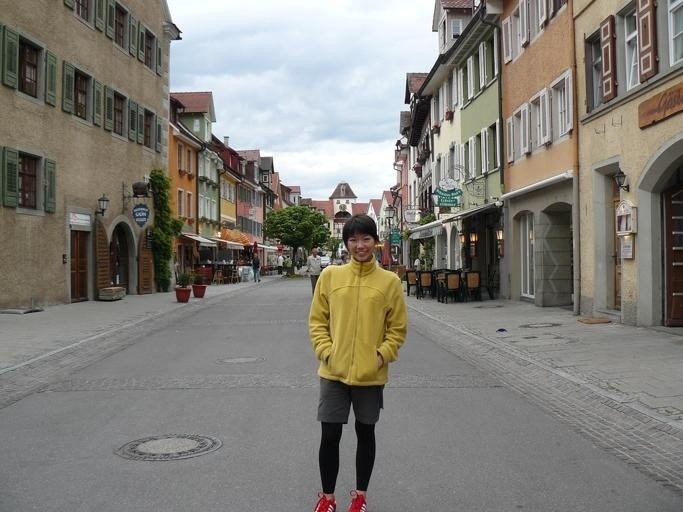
[314,495,336,512]
[347,493,367,512]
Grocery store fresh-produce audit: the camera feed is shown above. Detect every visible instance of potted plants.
[174,273,192,303]
[190,272,206,299]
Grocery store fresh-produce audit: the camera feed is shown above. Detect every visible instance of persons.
[251,250,350,284]
[413,256,421,270]
[306,246,321,294]
[306,212,409,512]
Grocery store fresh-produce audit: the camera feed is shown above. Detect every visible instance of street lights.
[391,190,403,263]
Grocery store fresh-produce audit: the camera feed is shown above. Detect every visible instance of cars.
[319,256,331,269]
[333,260,342,265]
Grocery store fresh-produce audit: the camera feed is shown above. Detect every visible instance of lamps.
[493,223,503,241]
[611,168,629,192]
[95,192,109,217]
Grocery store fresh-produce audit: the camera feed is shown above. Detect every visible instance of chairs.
[227,270,238,283]
[406,268,482,303]
[215,270,223,285]
